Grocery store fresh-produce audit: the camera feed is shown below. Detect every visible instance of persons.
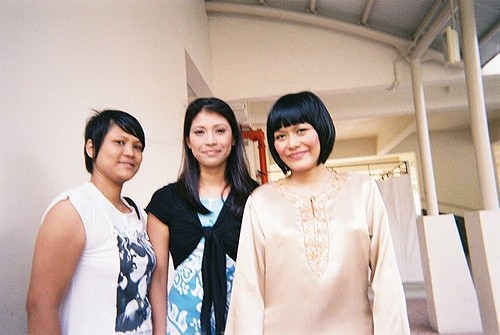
[27,110,156,335]
[145,98,261,335]
[223,92,409,335]
